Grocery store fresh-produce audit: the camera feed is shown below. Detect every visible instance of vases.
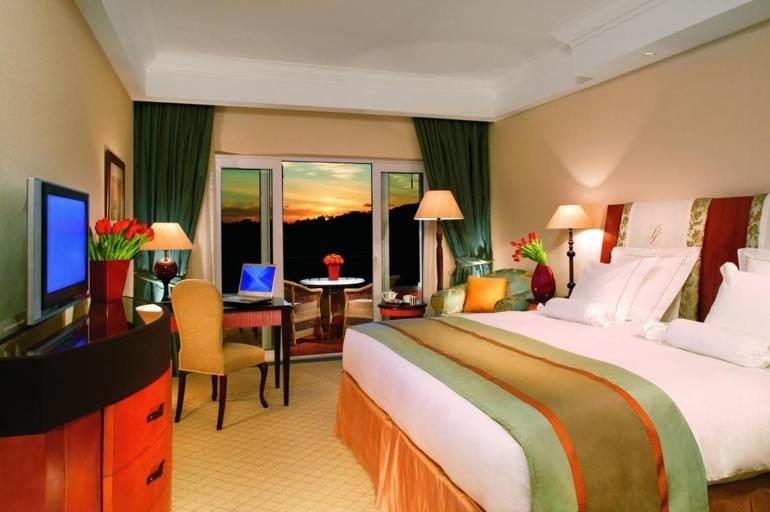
[88,258,133,304]
[530,261,557,301]
[327,263,343,281]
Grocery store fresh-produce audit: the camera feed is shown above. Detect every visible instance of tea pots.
[382,290,398,302]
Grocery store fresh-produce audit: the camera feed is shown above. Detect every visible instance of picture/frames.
[104,145,126,224]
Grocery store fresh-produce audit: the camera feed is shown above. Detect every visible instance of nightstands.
[526,296,548,311]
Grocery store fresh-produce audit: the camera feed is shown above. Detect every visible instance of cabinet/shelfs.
[0,291,173,512]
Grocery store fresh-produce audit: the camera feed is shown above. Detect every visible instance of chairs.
[282,279,325,347]
[429,267,535,317]
[342,274,403,341]
[168,277,272,433]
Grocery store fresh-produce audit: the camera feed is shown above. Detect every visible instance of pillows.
[460,275,511,314]
[537,240,770,372]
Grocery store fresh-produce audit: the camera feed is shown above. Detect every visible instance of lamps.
[544,202,596,296]
[411,189,465,288]
[138,220,196,301]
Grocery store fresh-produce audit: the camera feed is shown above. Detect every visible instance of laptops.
[223,263,277,305]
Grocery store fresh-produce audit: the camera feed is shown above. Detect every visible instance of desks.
[299,276,367,344]
[378,299,428,322]
[163,295,297,407]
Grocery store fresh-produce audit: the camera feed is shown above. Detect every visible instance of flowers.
[322,253,346,265]
[88,217,157,258]
[507,231,552,265]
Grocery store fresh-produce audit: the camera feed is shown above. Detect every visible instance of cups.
[410,296,416,304]
[403,295,412,302]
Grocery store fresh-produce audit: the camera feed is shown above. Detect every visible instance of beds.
[325,192,770,512]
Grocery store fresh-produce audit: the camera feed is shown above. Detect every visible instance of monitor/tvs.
[26,177,89,325]
[25,314,88,356]
